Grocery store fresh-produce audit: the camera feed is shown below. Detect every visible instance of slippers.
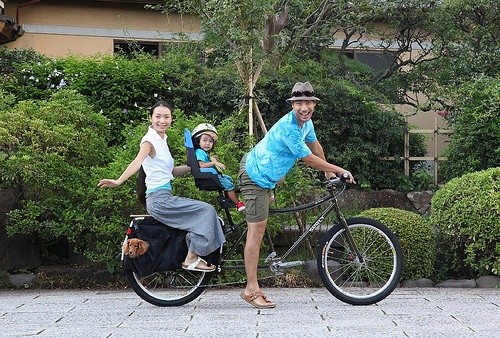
[182,256,216,272]
[240,290,276,309]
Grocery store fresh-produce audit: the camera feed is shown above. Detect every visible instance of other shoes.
[237,202,245,211]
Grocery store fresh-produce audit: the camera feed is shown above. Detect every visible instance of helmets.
[191,123,218,142]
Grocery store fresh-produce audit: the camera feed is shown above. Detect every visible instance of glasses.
[291,90,314,96]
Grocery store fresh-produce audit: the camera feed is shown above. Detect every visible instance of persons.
[97,99,226,271]
[237,81,357,307]
[191,122,245,212]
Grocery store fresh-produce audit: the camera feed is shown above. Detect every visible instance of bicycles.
[120,172,403,307]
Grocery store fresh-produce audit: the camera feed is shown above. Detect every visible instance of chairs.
[183,127,224,192]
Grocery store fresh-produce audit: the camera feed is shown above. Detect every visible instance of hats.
[286,81,322,104]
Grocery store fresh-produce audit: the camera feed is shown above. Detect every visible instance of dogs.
[124,238,149,258]
